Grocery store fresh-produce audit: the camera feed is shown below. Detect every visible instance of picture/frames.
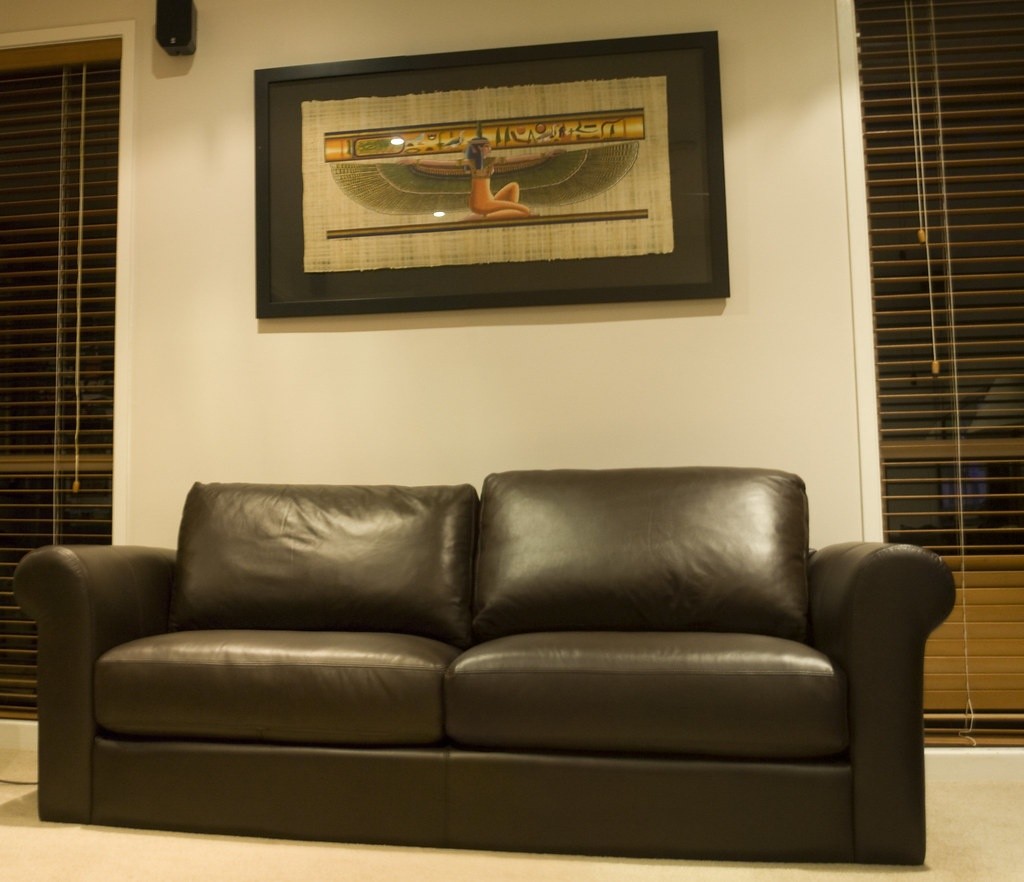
[253,31,730,319]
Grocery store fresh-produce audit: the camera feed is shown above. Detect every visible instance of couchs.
[12,467,957,866]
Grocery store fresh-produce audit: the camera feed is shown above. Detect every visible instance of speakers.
[156,0,197,56]
